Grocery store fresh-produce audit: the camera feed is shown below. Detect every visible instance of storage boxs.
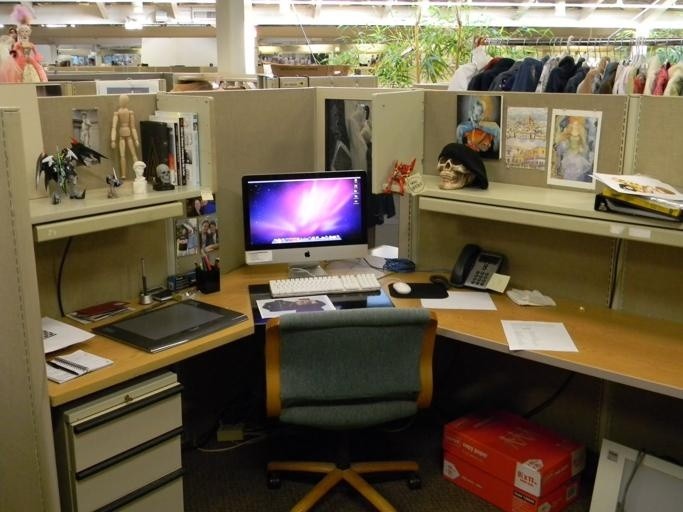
[444,408,585,512]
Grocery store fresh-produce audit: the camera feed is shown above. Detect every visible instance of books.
[588,170,683,224]
[141,109,199,191]
[44,349,113,386]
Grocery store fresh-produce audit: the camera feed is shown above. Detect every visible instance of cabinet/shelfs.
[0,86,683,512]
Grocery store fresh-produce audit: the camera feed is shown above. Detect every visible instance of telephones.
[449,244,505,291]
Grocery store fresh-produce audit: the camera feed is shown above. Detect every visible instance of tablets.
[91,300,249,354]
[268,273,381,298]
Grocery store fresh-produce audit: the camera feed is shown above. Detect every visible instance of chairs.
[264,309,438,512]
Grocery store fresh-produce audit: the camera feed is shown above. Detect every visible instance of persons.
[198,197,215,213]
[206,221,218,244]
[186,229,198,255]
[132,161,146,194]
[187,199,201,214]
[0,6,49,83]
[198,219,211,250]
[176,228,189,256]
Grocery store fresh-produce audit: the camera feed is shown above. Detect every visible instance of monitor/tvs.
[393,282,411,294]
[242,170,368,278]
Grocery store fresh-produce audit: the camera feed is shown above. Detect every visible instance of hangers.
[474,32,683,75]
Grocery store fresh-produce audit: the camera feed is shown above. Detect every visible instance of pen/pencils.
[46,361,78,375]
[140,259,146,295]
[194,256,220,271]
